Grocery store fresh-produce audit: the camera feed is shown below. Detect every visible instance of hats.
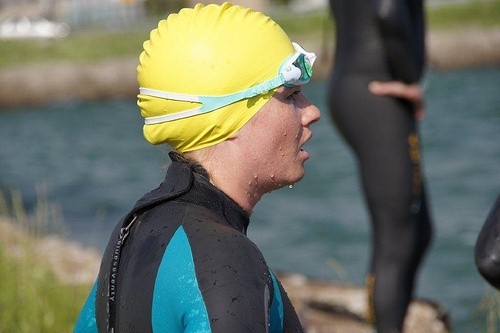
[136,2,317,155]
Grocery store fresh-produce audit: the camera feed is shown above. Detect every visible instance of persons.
[71,3,321,333]
[324,0,437,332]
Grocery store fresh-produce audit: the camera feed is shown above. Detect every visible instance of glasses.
[278,42,316,89]
